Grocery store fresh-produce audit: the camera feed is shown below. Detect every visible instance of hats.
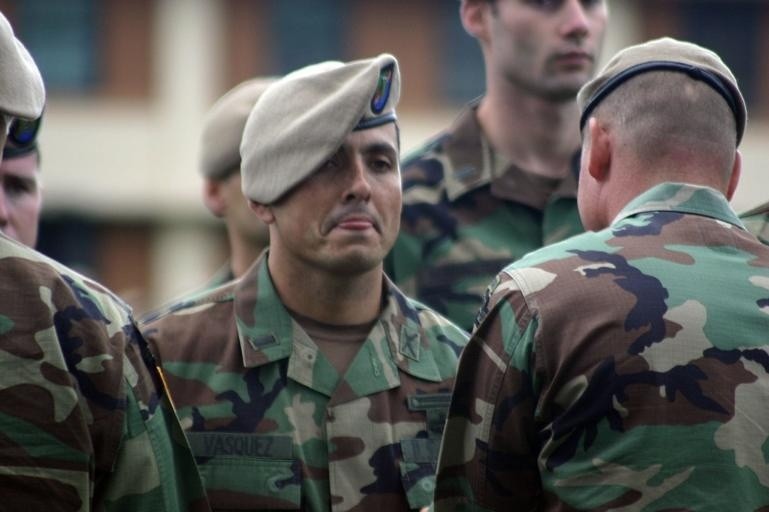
[238,53,401,206]
[200,79,282,180]
[0,9,46,148]
[576,37,747,149]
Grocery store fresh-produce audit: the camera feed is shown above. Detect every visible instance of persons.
[386,0,612,327]
[432,39,769,509]
[137,72,270,323]
[735,201,769,245]
[141,54,469,511]
[0,10,208,512]
[0,116,44,250]
